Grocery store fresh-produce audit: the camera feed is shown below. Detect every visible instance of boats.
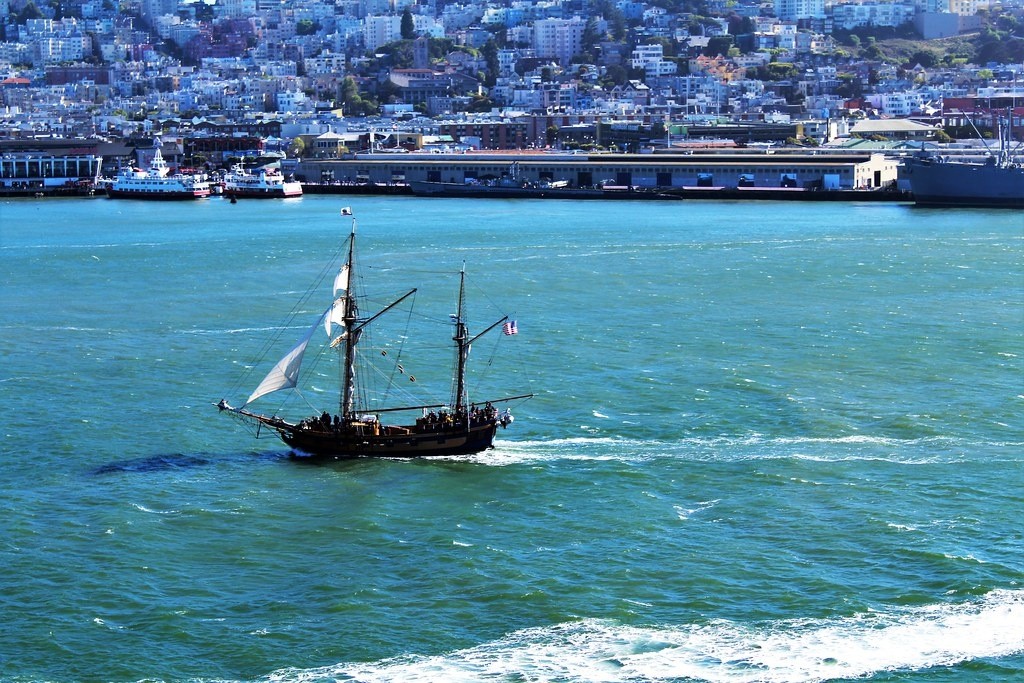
[410,181,682,203]
[0,153,109,198]
[211,155,302,198]
[100,141,211,201]
[211,219,536,463]
[903,107,1023,209]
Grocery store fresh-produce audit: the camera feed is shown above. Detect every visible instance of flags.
[502,320,518,335]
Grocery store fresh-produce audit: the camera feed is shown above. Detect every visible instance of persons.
[333,414,339,432]
[428,401,496,429]
[300,411,331,430]
[469,176,615,190]
